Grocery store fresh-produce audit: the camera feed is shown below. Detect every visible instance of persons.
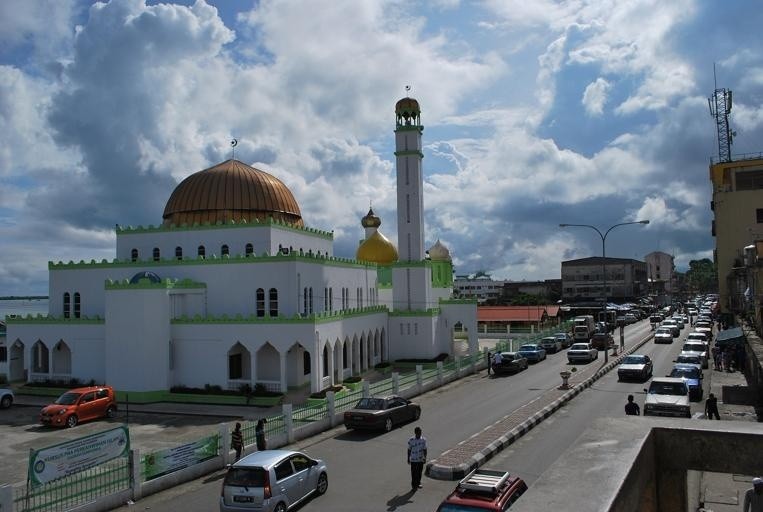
[255,420,265,451]
[704,393,721,420]
[494,351,504,377]
[230,423,244,461]
[408,427,427,490]
[625,395,640,415]
[487,353,493,376]
[716,319,732,332]
[743,477,763,512]
[712,341,745,373]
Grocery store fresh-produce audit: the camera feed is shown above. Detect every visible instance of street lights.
[555,218,649,362]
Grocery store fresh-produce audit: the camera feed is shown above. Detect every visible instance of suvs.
[38,385,118,429]
[436,468,525,512]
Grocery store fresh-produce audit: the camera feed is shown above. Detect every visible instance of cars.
[490,292,717,422]
[217,449,331,512]
[0,388,16,408]
[340,393,423,435]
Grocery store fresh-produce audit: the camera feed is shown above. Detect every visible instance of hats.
[753,477,762,485]
